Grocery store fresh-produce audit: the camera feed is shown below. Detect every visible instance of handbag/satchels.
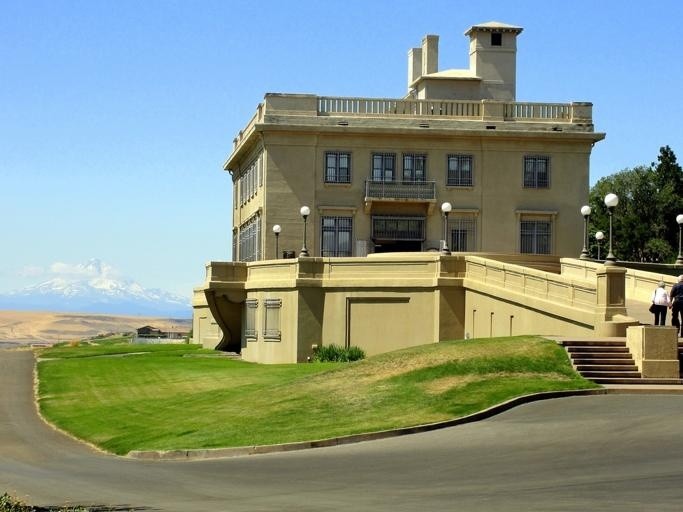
[649,304,656,313]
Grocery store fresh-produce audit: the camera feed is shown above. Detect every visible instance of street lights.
[442,202,452,256]
[273,224,282,260]
[580,193,619,266]
[300,206,311,257]
[676,214,683,264]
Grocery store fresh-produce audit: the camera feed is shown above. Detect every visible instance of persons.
[652,281,672,325]
[669,275,683,338]
